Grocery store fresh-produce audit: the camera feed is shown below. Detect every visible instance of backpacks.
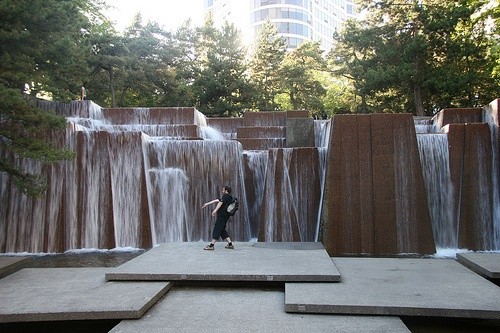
[226,194,239,216]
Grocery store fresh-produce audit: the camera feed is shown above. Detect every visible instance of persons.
[236,112,243,118]
[321,111,327,120]
[81,82,90,100]
[202,185,234,250]
[275,105,284,111]
[313,112,319,120]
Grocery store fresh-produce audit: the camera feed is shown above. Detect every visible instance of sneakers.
[225,245,234,249]
[203,245,214,250]
